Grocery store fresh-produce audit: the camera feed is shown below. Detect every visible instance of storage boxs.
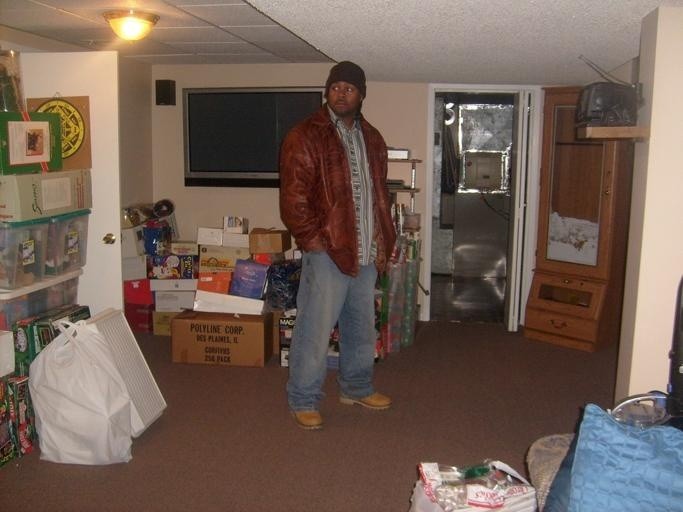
[35,305,91,350]
[146,255,193,279]
[199,246,251,272]
[1,270,83,330]
[122,221,147,256]
[172,242,199,256]
[14,302,79,377]
[197,271,234,295]
[151,311,179,336]
[0,111,62,174]
[123,278,154,304]
[125,303,154,333]
[122,254,149,280]
[46,209,93,277]
[155,291,195,312]
[0,168,94,222]
[223,215,248,233]
[248,229,293,253]
[171,310,272,367]
[0,218,50,289]
[223,232,249,248]
[150,278,198,291]
[198,226,223,246]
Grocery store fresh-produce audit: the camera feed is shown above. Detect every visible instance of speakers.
[155,79,176,106]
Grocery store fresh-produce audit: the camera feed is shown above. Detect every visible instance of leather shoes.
[339,389,392,410]
[288,405,325,430]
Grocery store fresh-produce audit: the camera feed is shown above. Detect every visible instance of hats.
[324,60,367,103]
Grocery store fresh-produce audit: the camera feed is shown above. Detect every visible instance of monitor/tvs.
[182,87,327,180]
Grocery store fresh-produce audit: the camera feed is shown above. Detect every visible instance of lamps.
[102,10,161,41]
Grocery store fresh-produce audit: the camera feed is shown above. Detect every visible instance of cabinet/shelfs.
[525,85,634,354]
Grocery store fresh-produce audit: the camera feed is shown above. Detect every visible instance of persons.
[278,60,398,432]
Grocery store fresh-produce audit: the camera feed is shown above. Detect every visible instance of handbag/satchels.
[440,124,460,196]
[539,390,683,512]
